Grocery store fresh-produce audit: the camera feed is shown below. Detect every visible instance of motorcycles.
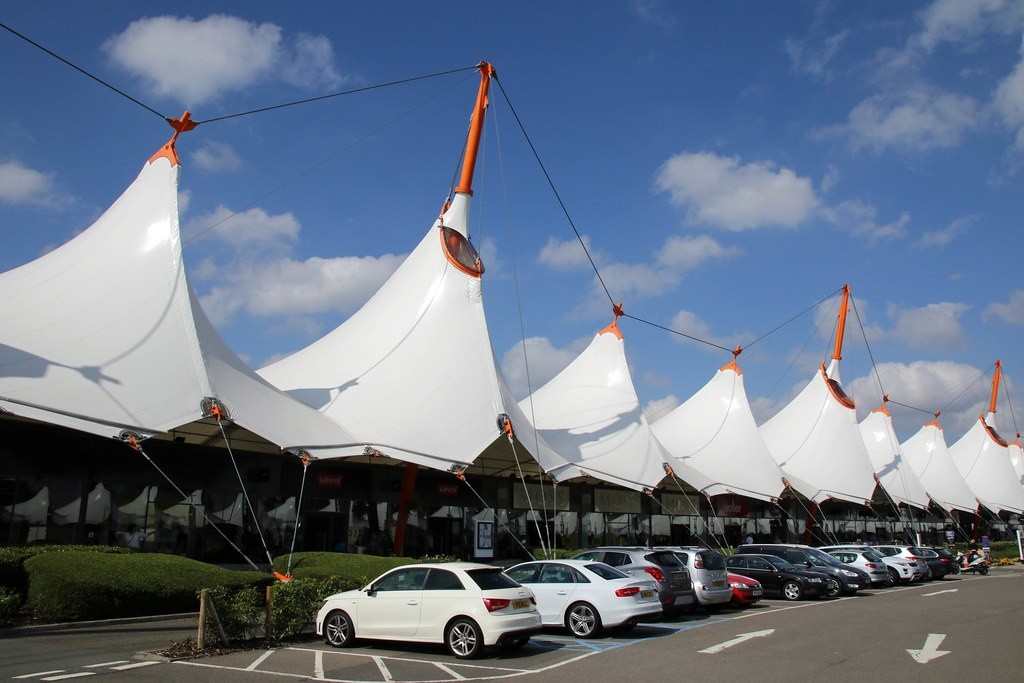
[954,550,989,575]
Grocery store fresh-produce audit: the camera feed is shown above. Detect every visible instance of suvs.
[639,548,733,609]
[571,546,696,618]
[733,543,868,597]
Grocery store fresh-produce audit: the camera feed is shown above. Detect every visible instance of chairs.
[846,555,852,563]
[542,568,560,583]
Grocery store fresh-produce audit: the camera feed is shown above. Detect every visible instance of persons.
[114,512,1024,568]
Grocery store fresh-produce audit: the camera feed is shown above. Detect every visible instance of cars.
[819,546,919,585]
[722,554,837,600]
[913,546,959,580]
[315,561,543,659]
[817,549,890,586]
[871,545,928,582]
[724,570,762,607]
[499,559,665,638]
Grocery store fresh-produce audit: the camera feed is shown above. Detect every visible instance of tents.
[648,343,837,548]
[517,301,734,562]
[756,283,918,548]
[1008,431,1024,493]
[898,407,1015,544]
[0,111,379,582]
[253,60,590,563]
[947,358,1024,523]
[858,395,976,550]
